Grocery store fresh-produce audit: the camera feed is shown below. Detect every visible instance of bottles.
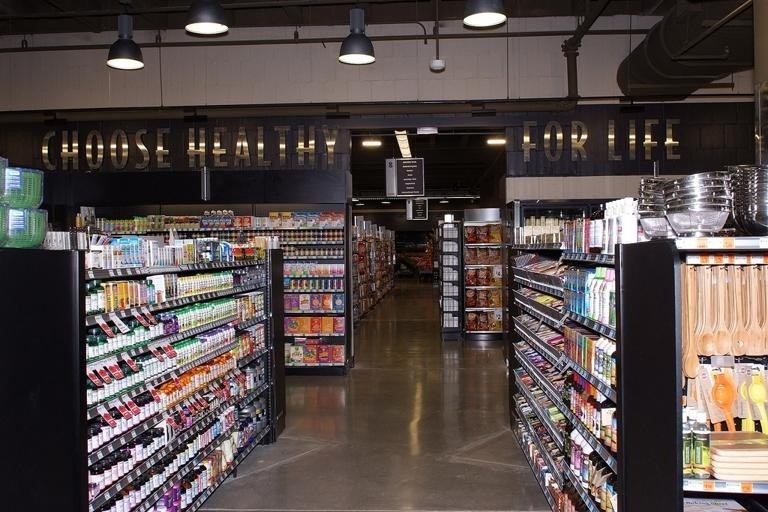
[76,211,345,259]
[85,267,266,511]
[683,404,713,479]
[562,197,637,512]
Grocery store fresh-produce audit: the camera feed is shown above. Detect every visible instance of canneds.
[290,279,344,289]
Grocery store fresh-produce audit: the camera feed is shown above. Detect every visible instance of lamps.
[463,0,507,28]
[185,0,229,35]
[106,0,144,71]
[338,3,376,65]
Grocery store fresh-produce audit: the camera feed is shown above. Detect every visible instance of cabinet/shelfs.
[433,220,462,341]
[352,223,394,325]
[77,207,353,375]
[510,210,767,512]
[0,233,285,510]
[464,216,506,336]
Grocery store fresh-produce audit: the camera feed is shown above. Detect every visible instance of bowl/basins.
[637,163,767,237]
